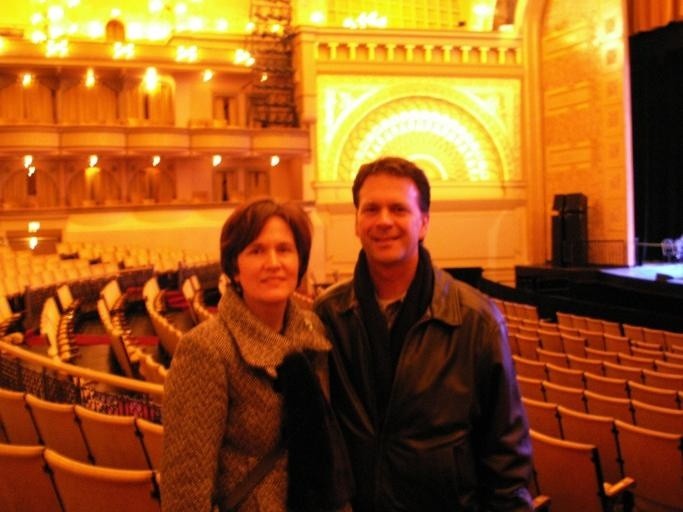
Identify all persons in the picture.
[311,157,533,512]
[159,199,335,512]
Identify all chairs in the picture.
[0,235,683,509]
